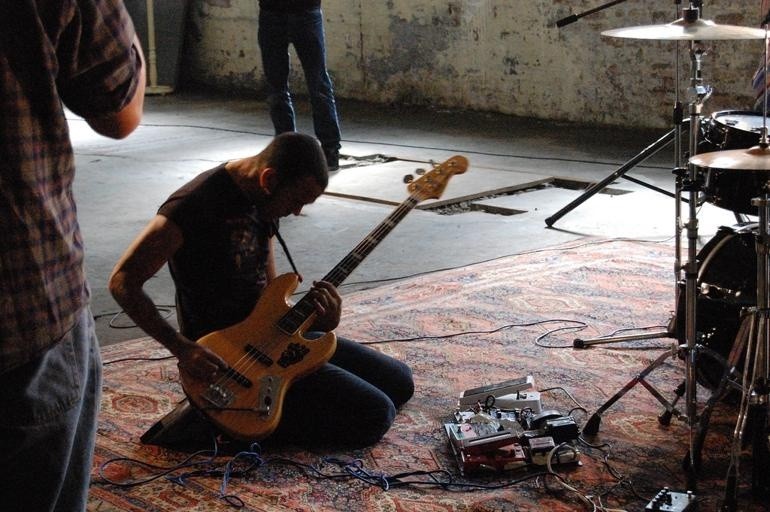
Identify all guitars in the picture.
[179,155,468,443]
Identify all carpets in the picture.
[93,233,769,510]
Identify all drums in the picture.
[667,222,770,412]
[696,110,770,216]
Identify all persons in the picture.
[255,0,342,172]
[109,132,415,452]
[0,0,149,512]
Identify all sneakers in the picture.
[139,393,191,448]
[324,149,340,171]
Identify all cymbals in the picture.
[600,18,769,40]
[688,145,770,170]
